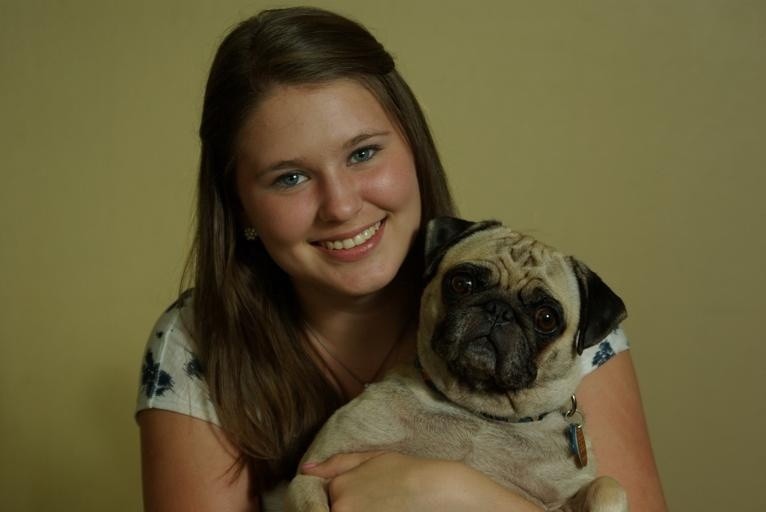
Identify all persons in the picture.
[133,7,669,512]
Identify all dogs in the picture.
[285,214,630,512]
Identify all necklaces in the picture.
[294,313,412,408]
